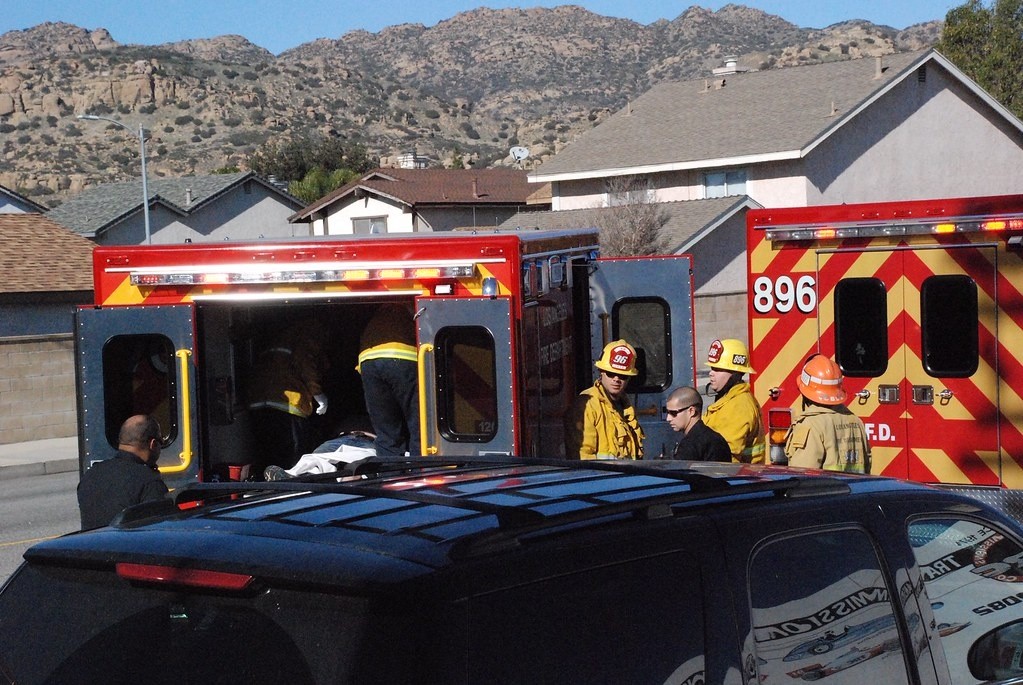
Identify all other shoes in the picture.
[264,465,295,482]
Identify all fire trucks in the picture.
[71,230,702,527]
[734,189,1022,499]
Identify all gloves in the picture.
[314,394,328,415]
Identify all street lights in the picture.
[75,113,152,246]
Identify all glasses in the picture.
[667,405,694,415]
[602,371,630,380]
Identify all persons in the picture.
[564,339,647,460]
[263,413,377,491]
[666,386,732,462]
[76,414,169,530]
[702,339,766,465]
[354,302,421,476]
[238,316,340,469]
[783,353,872,475]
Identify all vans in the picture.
[0,452,1022,685]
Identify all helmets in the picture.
[594,339,638,376]
[704,339,757,373]
[797,355,847,405]
